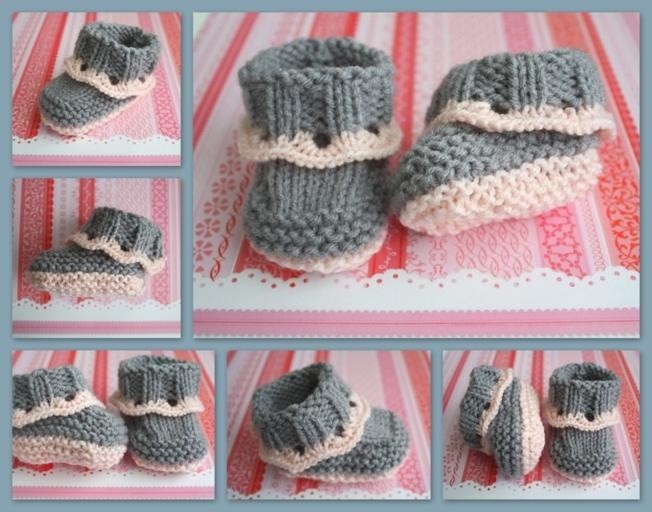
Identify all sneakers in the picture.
[13,366,129,470]
[233,36,403,273]
[545,362,621,484]
[36,23,160,137]
[460,366,547,479]
[29,206,165,296]
[392,50,617,236]
[251,362,413,484]
[109,355,210,472]
